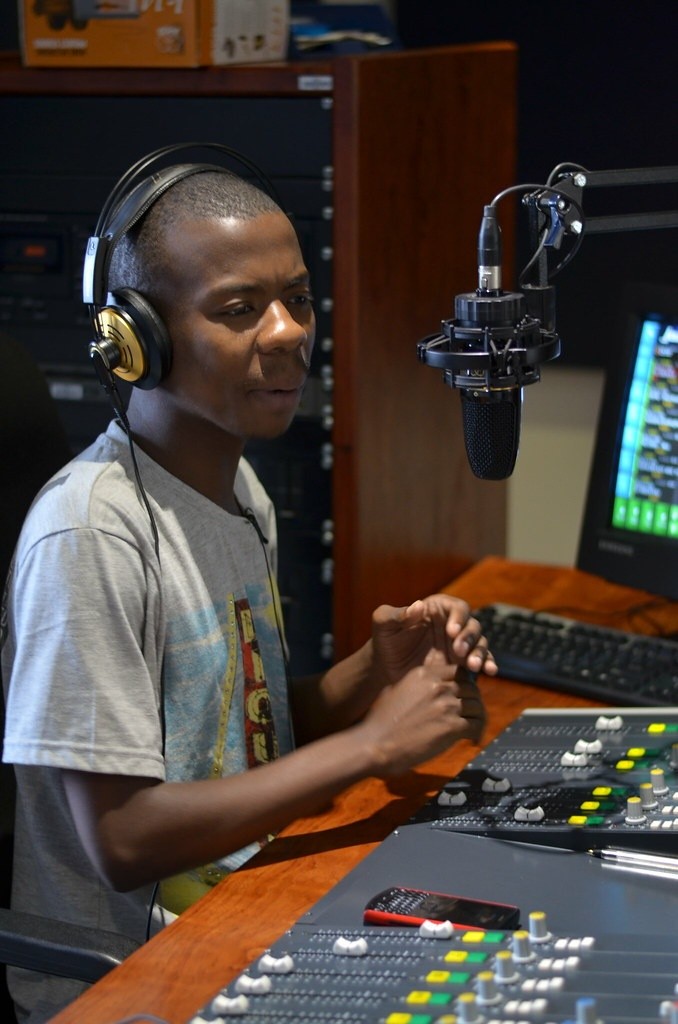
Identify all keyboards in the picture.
[469,602,678,709]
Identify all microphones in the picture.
[415,204,561,482]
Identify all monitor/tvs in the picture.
[576,286,678,599]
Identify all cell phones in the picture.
[364,884,521,934]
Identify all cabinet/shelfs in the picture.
[0,41,518,666]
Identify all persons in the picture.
[1,164,504,1024]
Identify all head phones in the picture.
[80,142,296,390]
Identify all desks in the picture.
[53,556,678,1024]
